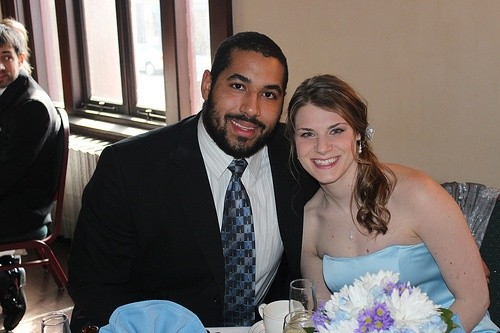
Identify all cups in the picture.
[40,313,71,333]
[258,300,305,333]
[283,310,318,333]
[289,279,315,319]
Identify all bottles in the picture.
[81,325,99,333]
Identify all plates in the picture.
[248,319,265,333]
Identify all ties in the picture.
[219,158,255,328]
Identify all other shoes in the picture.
[0,254,27,332]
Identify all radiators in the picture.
[49,132,114,239]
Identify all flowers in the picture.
[310,270,459,333]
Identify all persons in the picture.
[0,19,63,331]
[288,74,500,333]
[65,30,490,333]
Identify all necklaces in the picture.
[323,192,354,239]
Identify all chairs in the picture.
[0,100,70,287]
